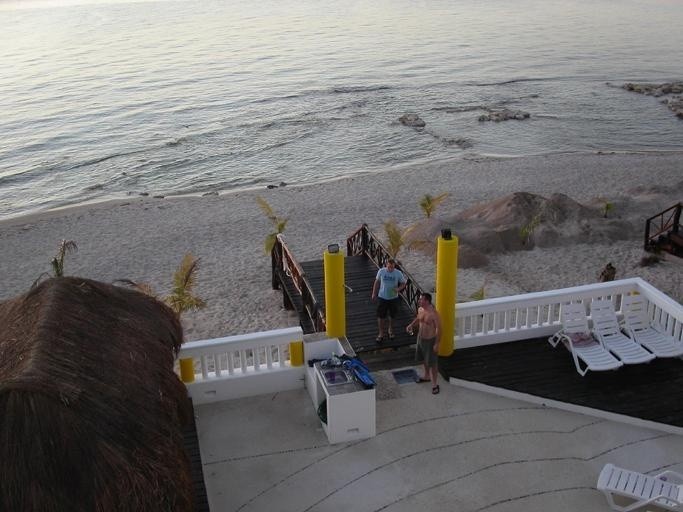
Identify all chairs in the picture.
[595,460,683,512]
[545,291,683,380]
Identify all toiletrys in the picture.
[332,351,339,365]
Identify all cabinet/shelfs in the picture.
[298,329,379,447]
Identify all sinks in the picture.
[320,367,355,386]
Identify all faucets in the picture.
[343,360,352,364]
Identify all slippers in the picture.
[388,328,395,340]
[431,384,440,395]
[376,335,384,344]
[415,377,431,383]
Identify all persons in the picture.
[370,258,406,342]
[405,292,441,394]
